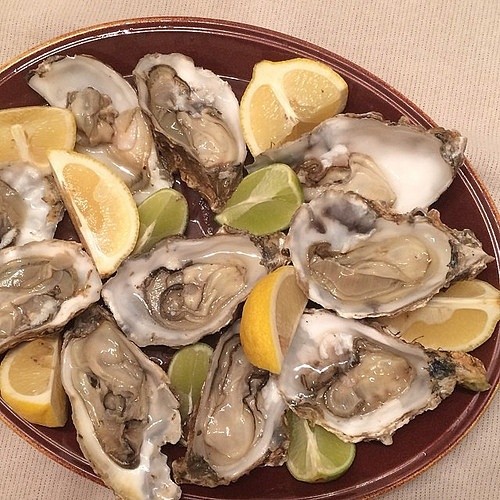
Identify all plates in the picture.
[1,15,500,500]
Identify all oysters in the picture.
[0,52,494,500]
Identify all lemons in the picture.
[0,105,77,179]
[167,342,219,445]
[239,57,349,159]
[376,277,500,353]
[285,409,356,483]
[1,337,67,427]
[214,163,305,236]
[130,188,190,258]
[240,265,308,373]
[46,147,140,279]
